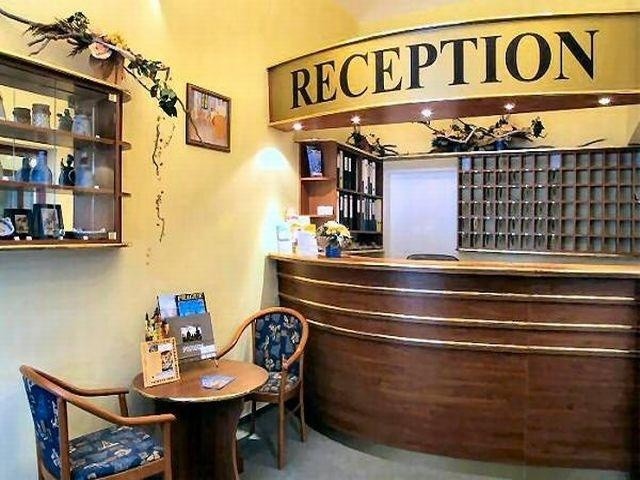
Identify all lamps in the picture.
[284,208,311,227]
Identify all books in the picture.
[159,291,216,364]
[337,148,376,231]
[307,144,323,177]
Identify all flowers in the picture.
[315,221,352,247]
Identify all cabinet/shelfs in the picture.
[458,146,640,259]
[0,48,131,252]
[298,140,385,252]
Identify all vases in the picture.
[326,245,340,257]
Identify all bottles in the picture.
[71,114,93,138]
[27,150,51,185]
[30,103,51,129]
[15,157,34,182]
[56,107,73,133]
[67,153,94,188]
[12,107,31,127]
[58,154,75,186]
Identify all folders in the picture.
[336,153,352,190]
[339,192,360,230]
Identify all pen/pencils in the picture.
[213,357,219,368]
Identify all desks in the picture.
[131,354,269,480]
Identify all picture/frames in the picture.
[34,202,64,240]
[186,82,232,151]
[300,143,326,178]
[5,207,33,241]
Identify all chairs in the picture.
[212,306,308,470]
[20,363,175,480]
[407,253,459,261]
